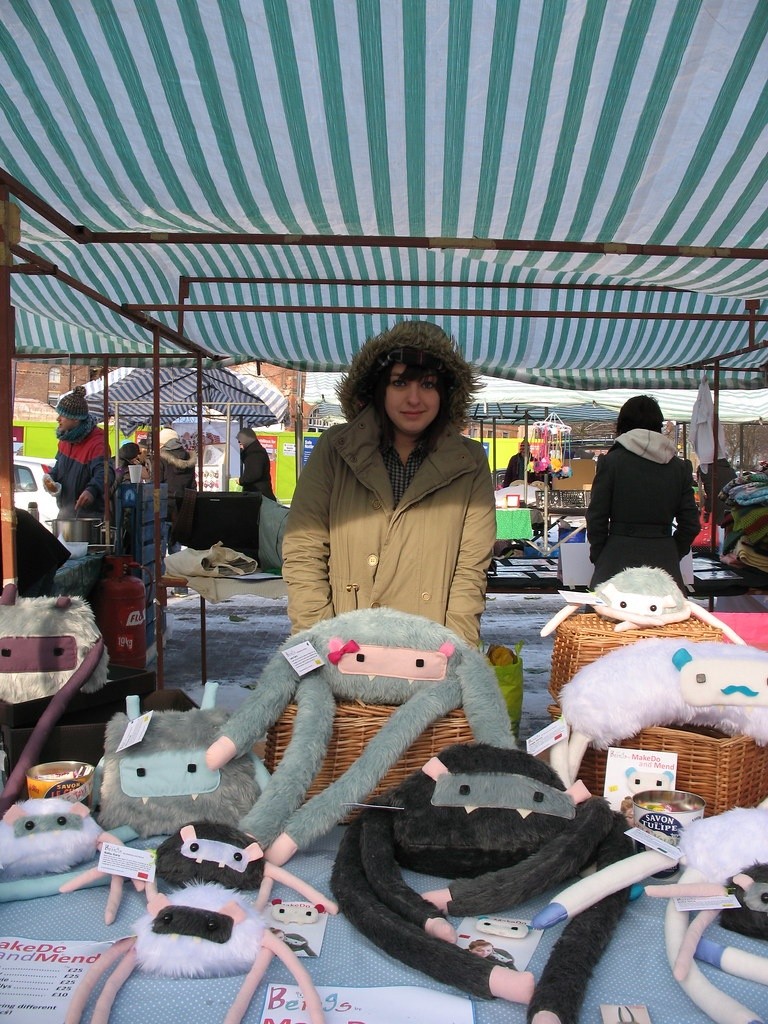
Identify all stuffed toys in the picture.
[0,566,768,1024]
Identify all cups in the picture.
[26,761,94,812]
[128,465,142,483]
[632,791,707,881]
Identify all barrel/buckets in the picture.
[558,527,586,543]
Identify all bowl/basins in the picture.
[62,541,89,558]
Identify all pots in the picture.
[45,517,105,544]
[100,526,127,554]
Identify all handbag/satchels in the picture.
[476,639,525,741]
[691,504,719,552]
[163,547,258,577]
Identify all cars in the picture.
[12,455,61,534]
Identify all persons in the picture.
[697,458,738,554]
[584,395,701,613]
[235,428,277,503]
[109,443,142,526]
[281,320,496,651]
[159,429,197,597]
[48,386,116,521]
[503,440,544,488]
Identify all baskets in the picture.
[544,704,768,819]
[536,489,592,516]
[264,697,478,824]
[548,612,722,706]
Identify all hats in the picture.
[119,442,141,462]
[55,386,90,420]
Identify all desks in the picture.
[161,553,768,684]
[539,508,588,517]
[0,822,768,1024]
[496,508,544,539]
[51,552,104,598]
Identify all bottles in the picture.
[27,502,39,522]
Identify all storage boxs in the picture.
[0,664,156,801]
